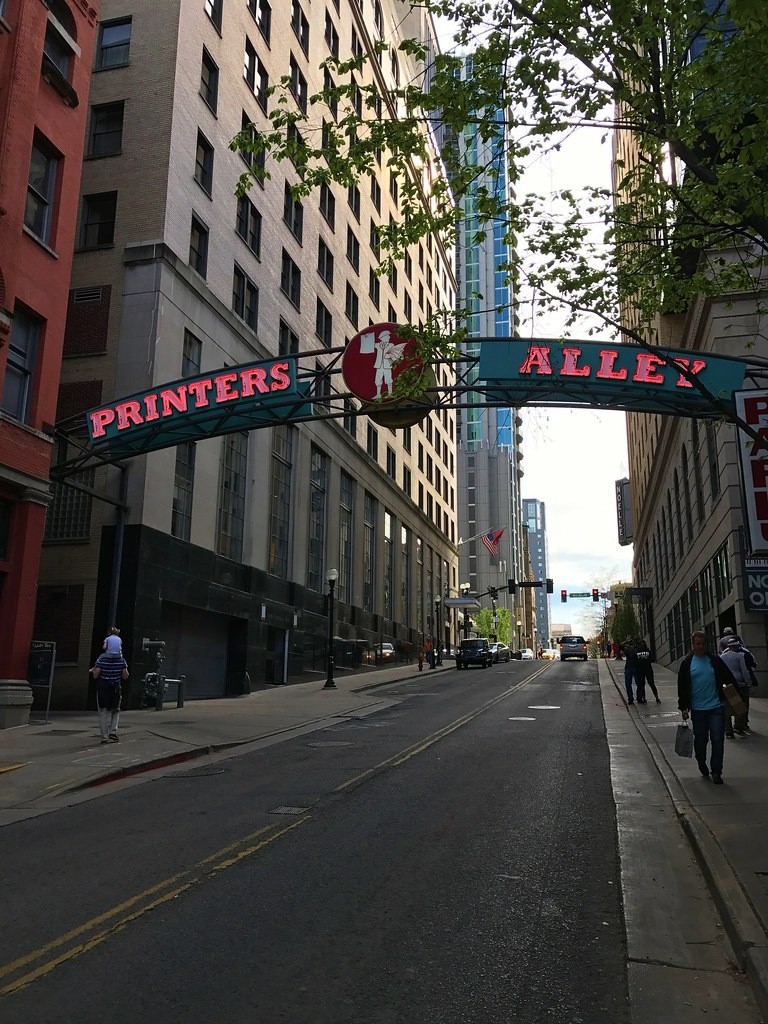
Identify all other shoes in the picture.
[628,700,633,705]
[742,728,752,736]
[100,741,107,744]
[638,700,643,703]
[107,734,119,743]
[698,760,709,777]
[643,699,647,705]
[656,699,661,704]
[726,732,735,739]
[89,668,93,672]
[710,771,724,784]
[733,728,746,736]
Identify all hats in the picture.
[723,627,734,635]
[727,638,742,646]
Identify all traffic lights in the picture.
[560,590,567,603]
[494,588,499,600]
[592,589,599,603]
[490,586,496,598]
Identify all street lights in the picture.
[459,582,470,640]
[322,567,339,691]
[517,621,522,650]
[534,628,539,660]
[434,594,443,667]
[613,600,624,660]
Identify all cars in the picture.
[542,649,560,660]
[376,643,395,660]
[521,648,533,660]
[488,642,511,664]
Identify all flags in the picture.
[482,531,498,557]
[493,530,504,545]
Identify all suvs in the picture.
[455,638,493,670]
[558,636,590,661]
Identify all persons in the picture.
[425,639,433,663]
[607,641,611,658]
[538,645,543,659]
[619,635,661,705]
[719,627,757,739]
[677,631,739,785]
[88,627,129,743]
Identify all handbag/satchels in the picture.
[675,719,693,758]
